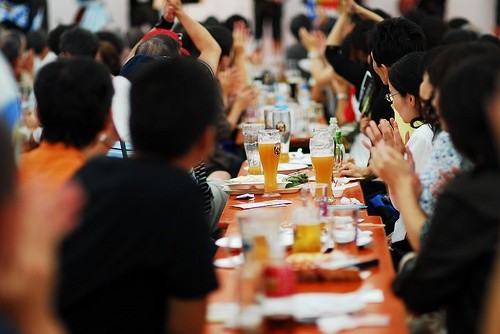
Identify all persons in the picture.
[0,0,500,334]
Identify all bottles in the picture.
[334,132,345,167]
[246,80,312,134]
[222,174,292,195]
[243,236,297,327]
[329,116,341,153]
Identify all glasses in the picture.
[385,92,401,102]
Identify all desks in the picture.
[234,107,328,147]
[218,159,369,228]
[211,215,410,334]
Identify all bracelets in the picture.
[155,15,175,29]
[308,50,318,58]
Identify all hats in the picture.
[143,29,189,56]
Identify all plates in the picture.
[215,235,242,250]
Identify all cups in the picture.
[237,108,357,260]
